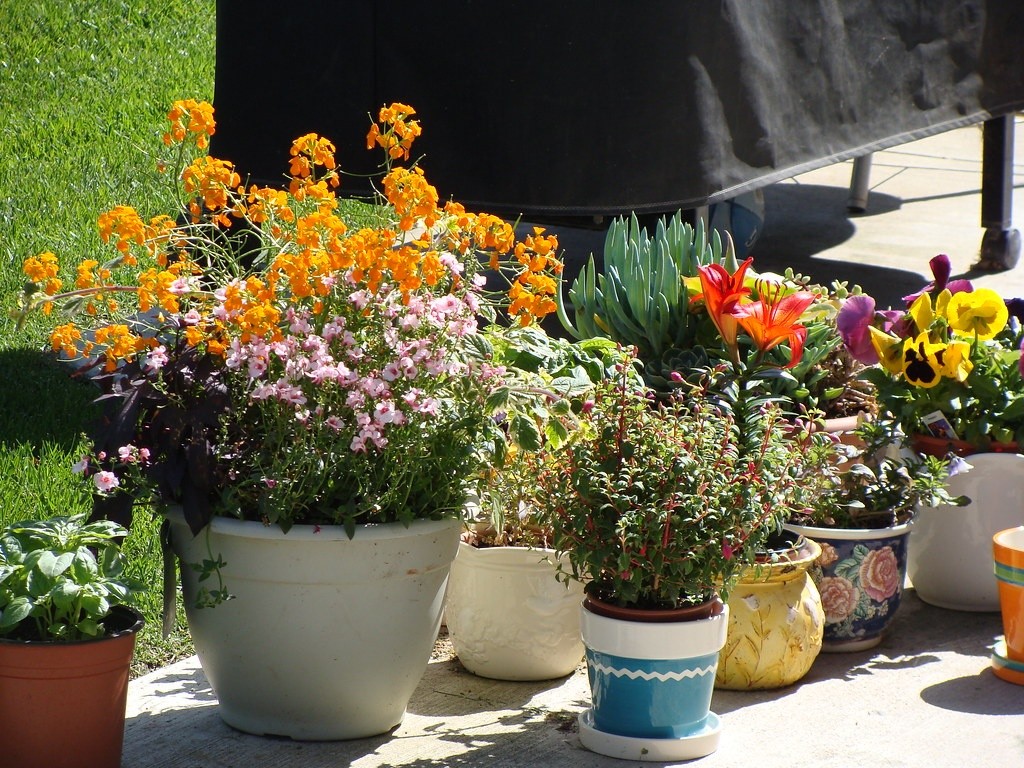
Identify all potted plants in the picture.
[443,328,644,685]
[0,512,145,768]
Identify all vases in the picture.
[785,527,918,654]
[575,595,729,762]
[713,528,825,692]
[905,454,1024,612]
[990,524,1024,685]
[161,508,464,739]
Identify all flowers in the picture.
[836,253,1024,450]
[671,257,821,544]
[516,343,841,604]
[22,96,561,537]
[849,421,978,525]
